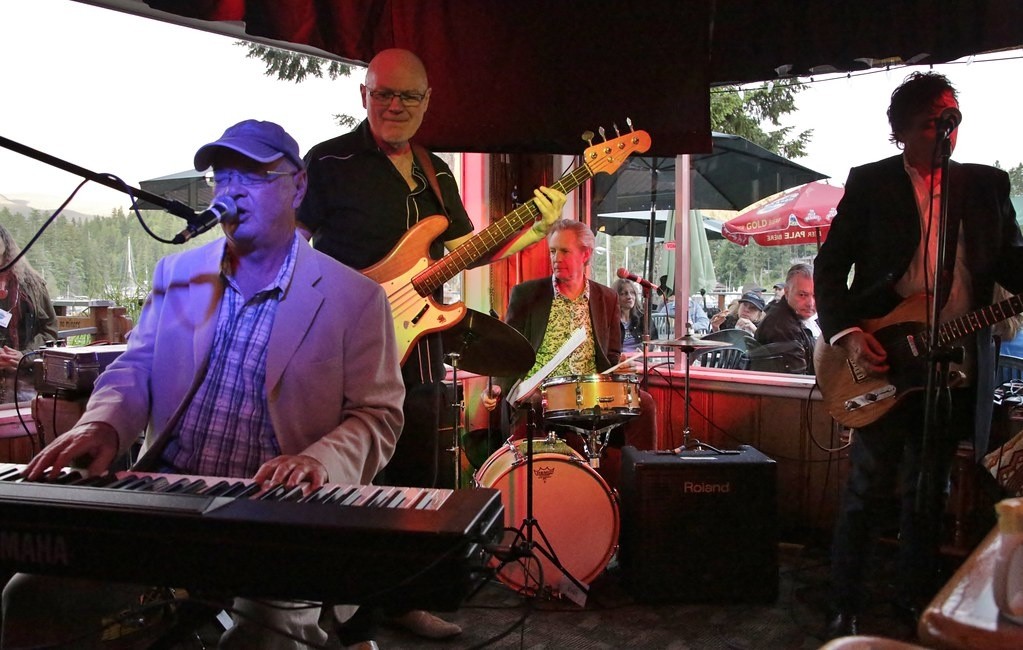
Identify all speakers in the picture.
[618,444,779,602]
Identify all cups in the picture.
[993,499,1023,621]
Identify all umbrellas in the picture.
[657,209,716,299]
[722,178,848,253]
[561,132,831,335]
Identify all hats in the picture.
[194,118,305,172]
[773,283,784,289]
[753,288,766,292]
[738,291,765,312]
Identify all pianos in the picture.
[0,459,507,650]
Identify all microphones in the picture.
[936,107,962,140]
[617,268,662,291]
[172,194,237,245]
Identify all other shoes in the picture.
[338,638,379,650]
[390,606,463,642]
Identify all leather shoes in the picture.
[822,589,859,644]
[889,593,927,633]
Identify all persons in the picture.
[709,281,787,338]
[659,300,710,333]
[610,278,659,351]
[745,264,817,375]
[814,72,1022,642]
[295,47,567,650]
[481,219,656,452]
[0,226,58,403]
[0,119,407,650]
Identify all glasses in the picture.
[365,85,425,107]
[204,165,298,187]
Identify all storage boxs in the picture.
[42,344,130,389]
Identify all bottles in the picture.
[652,346,661,364]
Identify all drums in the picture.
[540,371,643,421]
[470,432,623,595]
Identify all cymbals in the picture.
[643,337,734,349]
[420,303,537,378]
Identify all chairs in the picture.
[651,308,787,373]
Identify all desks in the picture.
[917,518,1023,650]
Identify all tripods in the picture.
[464,327,605,610]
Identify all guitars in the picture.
[813,293,1023,428]
[358,117,652,368]
